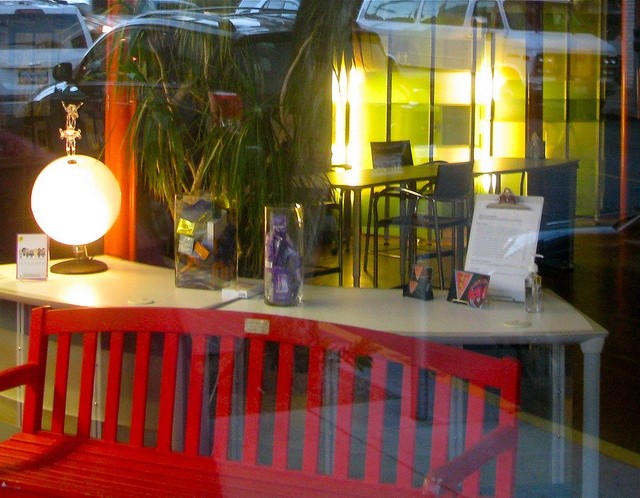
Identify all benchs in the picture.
[1,306,522,496]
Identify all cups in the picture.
[171,190,240,291]
[262,202,305,306]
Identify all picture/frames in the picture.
[16,234,49,280]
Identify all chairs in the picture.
[363,140,433,274]
[373,186,468,290]
[304,189,345,286]
[412,161,475,281]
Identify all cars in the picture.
[234,1,299,17]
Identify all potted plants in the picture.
[85,0,363,355]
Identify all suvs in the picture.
[23,6,404,190]
[0,0,93,123]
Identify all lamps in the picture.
[31,101,122,275]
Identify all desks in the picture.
[292,158,582,288]
[0,255,610,498]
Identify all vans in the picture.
[356,0,621,122]
